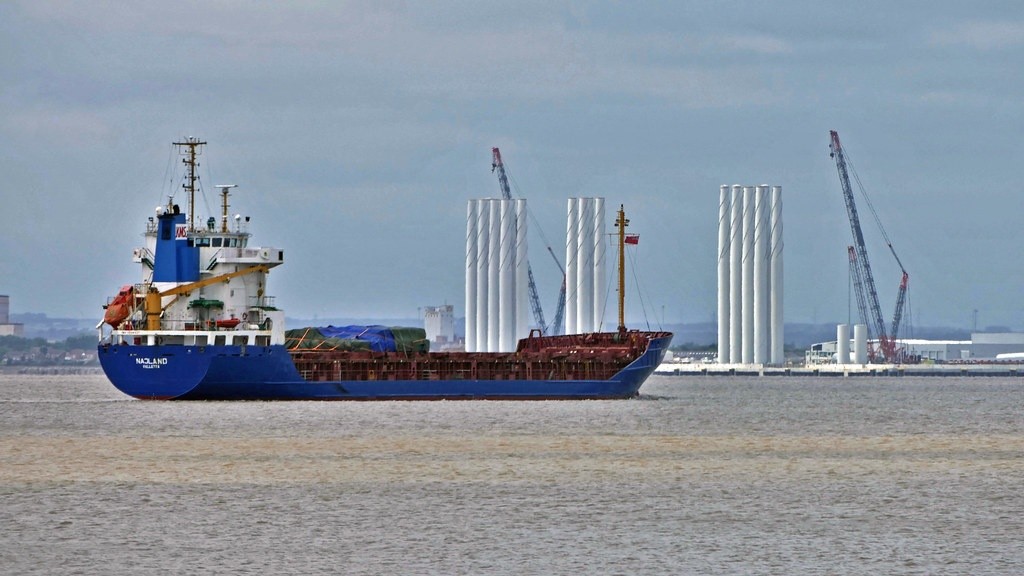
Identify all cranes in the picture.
[489,146,567,337]
[829,130,909,359]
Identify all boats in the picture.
[96,135,674,400]
